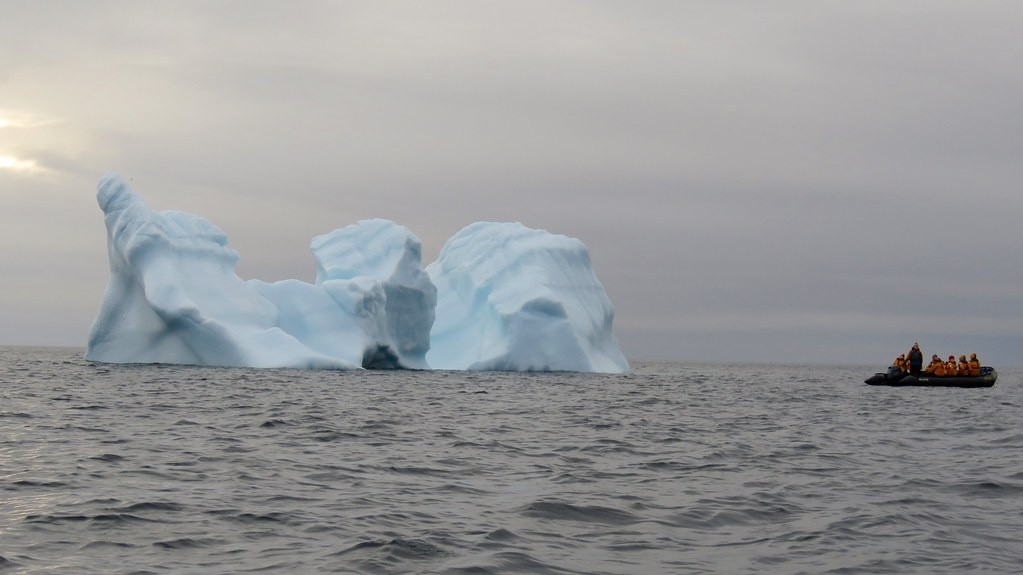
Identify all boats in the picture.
[864,366,998,388]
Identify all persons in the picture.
[925,354,981,377]
[902,342,923,377]
[893,354,905,373]
